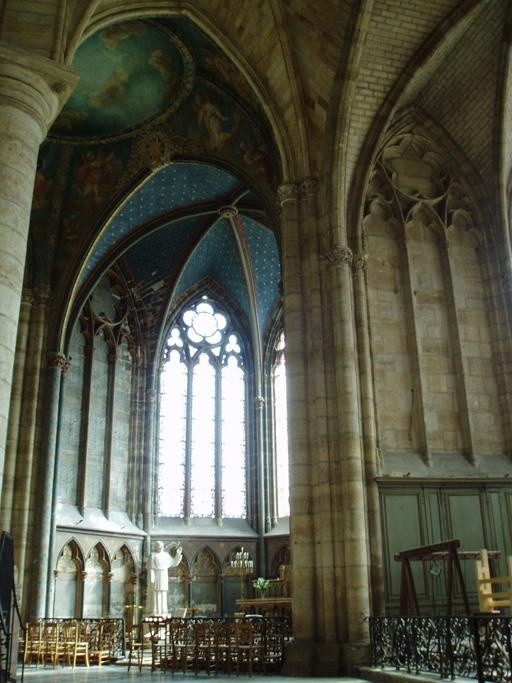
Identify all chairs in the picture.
[22,619,117,669]
[127,617,254,677]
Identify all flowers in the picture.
[252,577,272,593]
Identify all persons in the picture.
[148,541,183,613]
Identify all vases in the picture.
[260,588,266,600]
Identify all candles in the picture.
[230,546,254,568]
